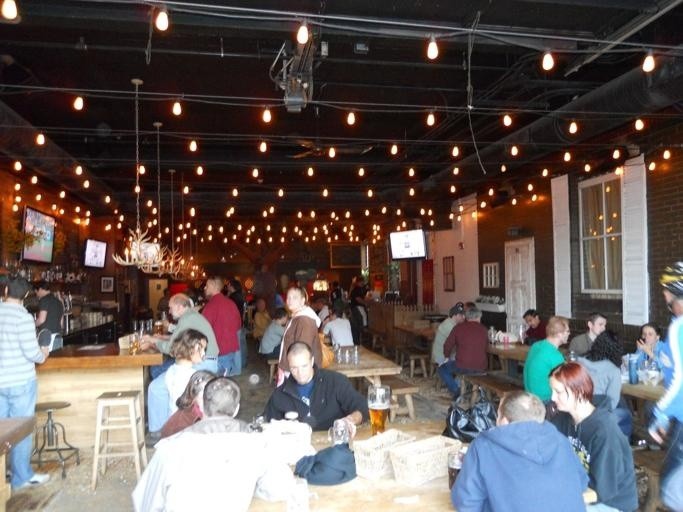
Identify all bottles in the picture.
[567,350,576,362]
[56,289,71,312]
[641,360,660,389]
[161,312,169,336]
[128,318,154,352]
[333,343,360,364]
[489,326,495,344]
[2,256,64,281]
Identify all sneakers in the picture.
[16,474,50,490]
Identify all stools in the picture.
[34,402,79,478]
[90,391,147,490]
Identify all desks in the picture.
[0,417,39,512]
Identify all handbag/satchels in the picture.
[442,387,496,442]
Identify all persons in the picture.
[132,378,295,512]
[0,276,49,491]
[647,260,683,512]
[136,276,374,446]
[32,282,64,352]
[432,301,664,511]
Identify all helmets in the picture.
[658,260,683,295]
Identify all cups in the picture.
[325,421,349,444]
[629,358,638,384]
[284,411,299,422]
[516,322,526,345]
[447,450,463,489]
[155,320,163,335]
[503,336,508,343]
[367,385,389,435]
[252,412,267,433]
[80,311,113,323]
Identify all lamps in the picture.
[642,50,655,73]
[296,24,309,45]
[426,39,438,60]
[1,1,18,22]
[155,11,170,32]
[0,92,671,276]
[542,53,555,71]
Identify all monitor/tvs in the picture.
[81,237,108,269]
[19,204,58,266]
[330,243,362,269]
[389,229,427,260]
[130,240,163,268]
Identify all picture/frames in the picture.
[101,277,114,293]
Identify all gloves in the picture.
[646,414,670,450]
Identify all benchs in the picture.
[255,324,683,511]
